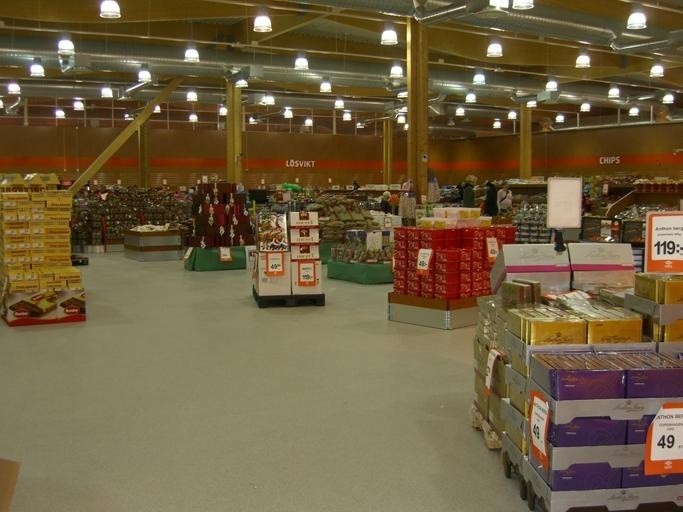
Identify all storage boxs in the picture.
[393,226,516,299]
[0,190,83,292]
[532,350,683,492]
[472,274,683,456]
[418,207,492,229]
[184,182,254,248]
[487,244,637,294]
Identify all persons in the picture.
[496,181,513,212]
[486,182,498,216]
[463,174,478,207]
[388,192,398,216]
[380,191,391,214]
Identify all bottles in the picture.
[664,261,672,281]
